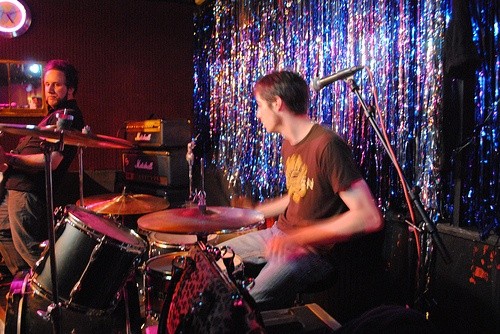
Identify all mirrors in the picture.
[0,59,48,117]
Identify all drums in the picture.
[29,205,147,318]
[142,252,243,321]
[147,229,219,257]
[4,270,131,334]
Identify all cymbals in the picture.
[77,192,170,214]
[137,205,267,234]
[0,123,129,150]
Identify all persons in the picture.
[214,71,383,301]
[0,60,88,274]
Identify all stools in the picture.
[281,270,333,305]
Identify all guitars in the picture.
[0,150,20,204]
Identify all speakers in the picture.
[121,117,191,209]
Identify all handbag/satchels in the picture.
[156,245,264,334]
[167,240,264,334]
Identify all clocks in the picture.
[0,0,32,38]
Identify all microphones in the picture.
[309,66,363,91]
